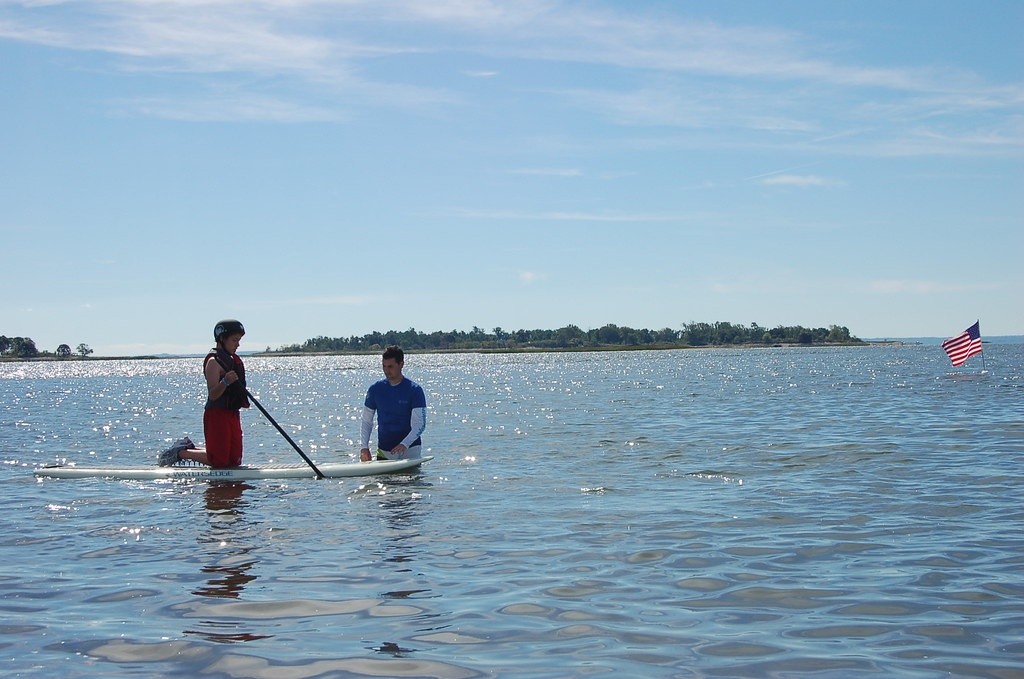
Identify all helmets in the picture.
[214,320,245,341]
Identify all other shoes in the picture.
[170,436,195,449]
[158,445,187,467]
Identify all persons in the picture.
[159,318,251,468]
[361,346,426,461]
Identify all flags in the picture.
[943,322,982,367]
